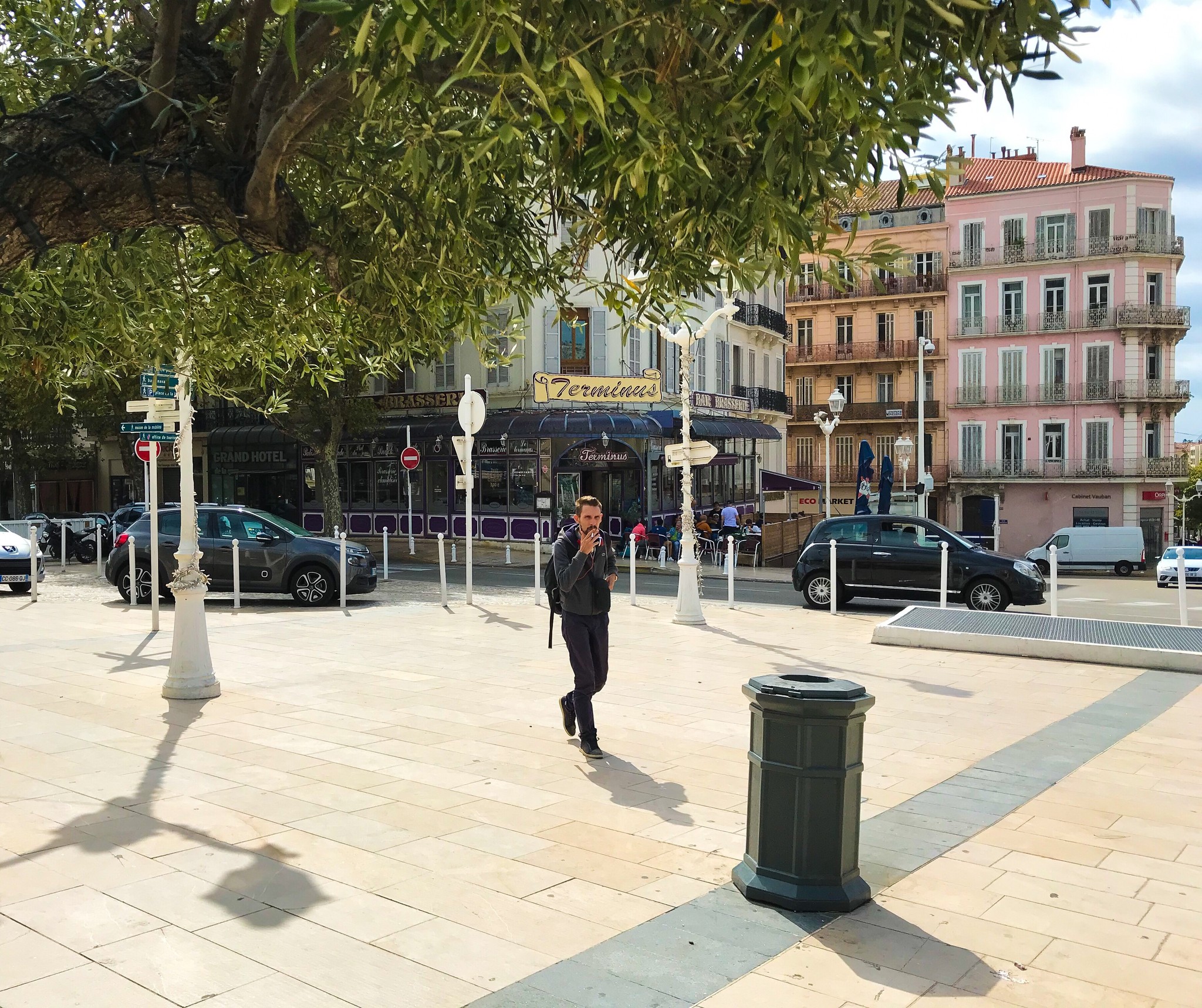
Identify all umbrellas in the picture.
[854,440,875,541]
[877,456,894,516]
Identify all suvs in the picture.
[791,514,1048,613]
[19,502,269,556]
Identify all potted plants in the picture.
[732,533,761,565]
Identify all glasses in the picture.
[714,517,719,518]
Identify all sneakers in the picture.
[579,738,604,758]
[559,696,575,737]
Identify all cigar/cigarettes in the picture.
[591,533,593,538]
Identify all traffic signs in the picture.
[121,423,164,432]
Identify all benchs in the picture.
[827,530,856,541]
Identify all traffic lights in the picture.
[131,439,143,467]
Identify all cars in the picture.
[105,506,377,607]
[1154,546,1202,588]
[0,523,45,593]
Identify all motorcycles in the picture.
[37,517,97,565]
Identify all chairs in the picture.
[886,530,899,546]
[622,521,773,569]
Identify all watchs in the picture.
[614,574,617,582]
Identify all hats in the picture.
[727,500,733,504]
[713,514,719,516]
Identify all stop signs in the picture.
[134,438,161,462]
[401,447,419,470]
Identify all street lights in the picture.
[621,251,744,626]
[1165,478,1202,547]
[812,388,846,516]
[894,437,915,496]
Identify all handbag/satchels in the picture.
[590,578,611,612]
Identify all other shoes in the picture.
[667,557,674,562]
[640,555,645,559]
[648,556,657,560]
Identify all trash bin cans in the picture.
[733,673,872,914]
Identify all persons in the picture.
[882,527,920,546]
[791,510,805,519]
[1197,536,1202,545]
[553,495,618,757]
[632,500,767,566]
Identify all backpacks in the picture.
[544,531,612,615]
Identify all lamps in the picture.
[436,434,444,449]
[602,431,609,448]
[499,433,508,447]
[371,437,379,450]
[756,453,762,464]
[737,453,743,464]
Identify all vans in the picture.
[1024,527,1146,577]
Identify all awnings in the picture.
[207,409,663,445]
[674,417,783,440]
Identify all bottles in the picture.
[746,526,748,534]
